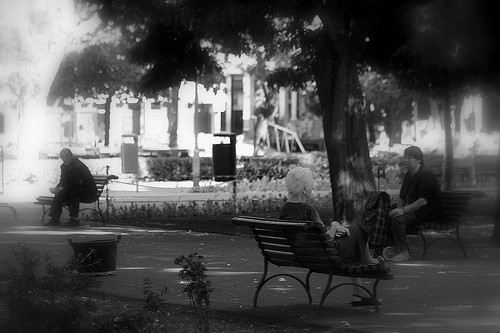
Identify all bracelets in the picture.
[402,206,406,214]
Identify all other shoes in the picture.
[43,219,61,226]
[63,218,81,226]
[391,251,410,262]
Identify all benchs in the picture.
[142,148,205,158]
[35,174,119,225]
[405,189,486,264]
[231,215,392,317]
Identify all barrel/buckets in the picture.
[68,232,123,277]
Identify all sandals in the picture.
[350,293,382,307]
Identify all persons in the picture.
[279,166,380,268]
[44,148,98,227]
[385,145,444,262]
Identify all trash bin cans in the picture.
[211,131,237,182]
[120,132,139,173]
